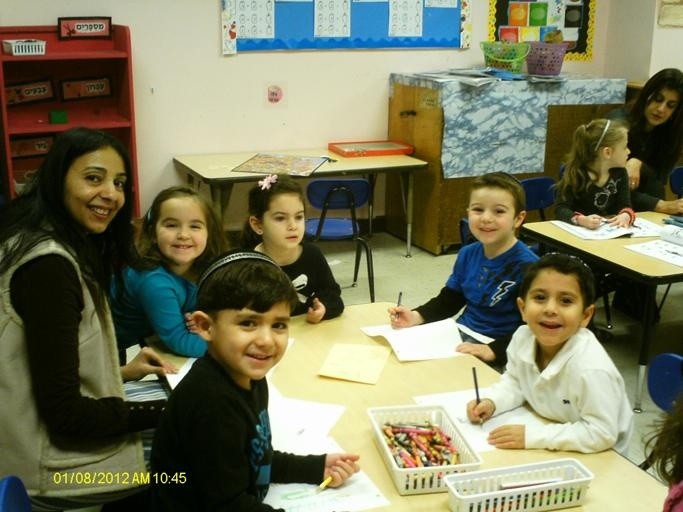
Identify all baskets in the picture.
[523,40,569,75]
[479,40,530,73]
[366,405,480,496]
[442,458,594,512]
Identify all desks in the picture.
[169,140,432,260]
[141,300,670,511]
[518,208,683,415]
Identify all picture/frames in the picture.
[325,138,419,160]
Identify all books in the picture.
[550,216,660,240]
[623,224,683,268]
[413,66,567,88]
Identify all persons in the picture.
[109,184,230,360]
[1,123,178,511]
[465,250,645,461]
[552,117,636,229]
[242,171,345,324]
[149,247,363,512]
[642,391,683,512]
[606,67,683,215]
[387,170,540,367]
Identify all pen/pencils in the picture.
[470,478,591,512]
[383,421,465,480]
[472,367,483,428]
[396,292,402,320]
[315,477,332,493]
[574,211,609,224]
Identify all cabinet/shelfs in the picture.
[382,67,632,259]
[0,20,142,246]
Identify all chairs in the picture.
[0,476,34,512]
[634,351,683,473]
[509,177,613,332]
[656,166,683,316]
[458,215,479,250]
[295,178,375,303]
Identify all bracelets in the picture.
[618,207,635,225]
[571,210,584,225]
